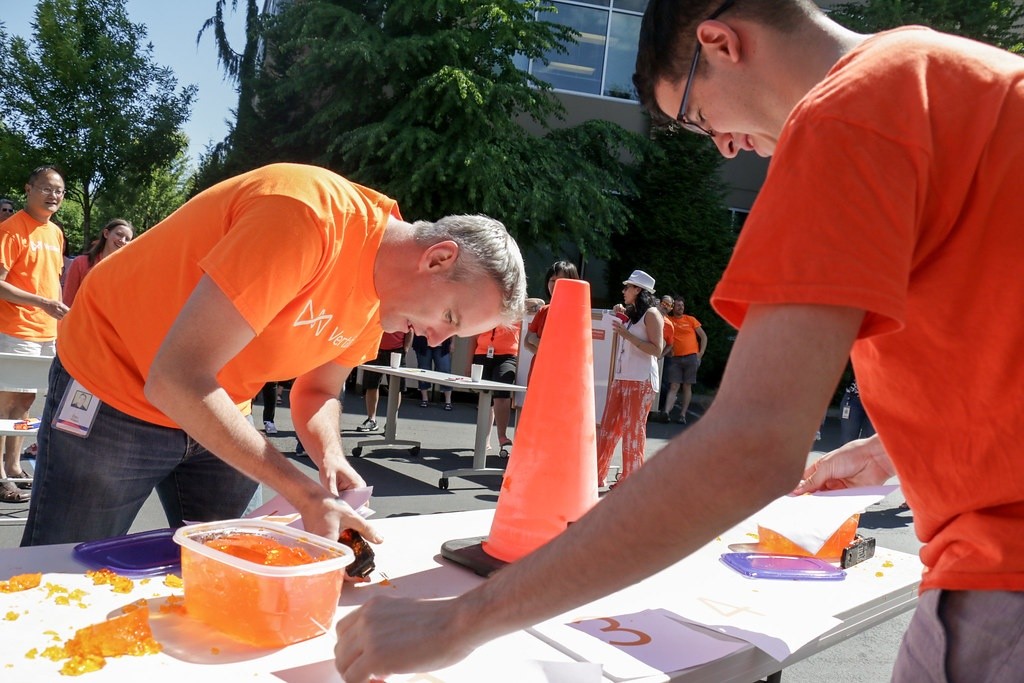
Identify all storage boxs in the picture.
[173,519,356,647]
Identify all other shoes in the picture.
[445,403,453,412]
[275,393,282,403]
[421,400,429,409]
[499,436,513,446]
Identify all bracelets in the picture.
[697,353,703,358]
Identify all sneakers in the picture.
[264,421,277,433]
[356,418,378,432]
[678,414,688,424]
[659,412,671,424]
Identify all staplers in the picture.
[338,529,375,578]
[840,533,875,569]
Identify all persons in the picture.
[649,293,707,425]
[597,266,666,491]
[523,260,579,391]
[24,218,140,460]
[334,0,1024,683]
[19,162,527,550]
[0,164,72,503]
[262,329,456,437]
[839,378,876,448]
[0,199,14,222]
[71,393,88,411]
[465,298,546,451]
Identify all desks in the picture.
[0,511,925,683]
[352,365,528,490]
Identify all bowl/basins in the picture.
[172,518,356,650]
[759,513,859,562]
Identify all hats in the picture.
[623,270,656,294]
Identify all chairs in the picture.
[0,353,55,524]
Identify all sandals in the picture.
[7,469,34,489]
[0,481,30,501]
[24,444,39,454]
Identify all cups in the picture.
[390,352,402,369]
[471,364,483,382]
[616,312,629,326]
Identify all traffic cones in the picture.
[439,278,599,578]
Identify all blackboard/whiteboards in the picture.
[511,308,618,428]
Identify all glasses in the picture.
[668,0,736,136]
[29,183,66,197]
[0,207,13,214]
[662,301,673,308]
[624,286,638,290]
